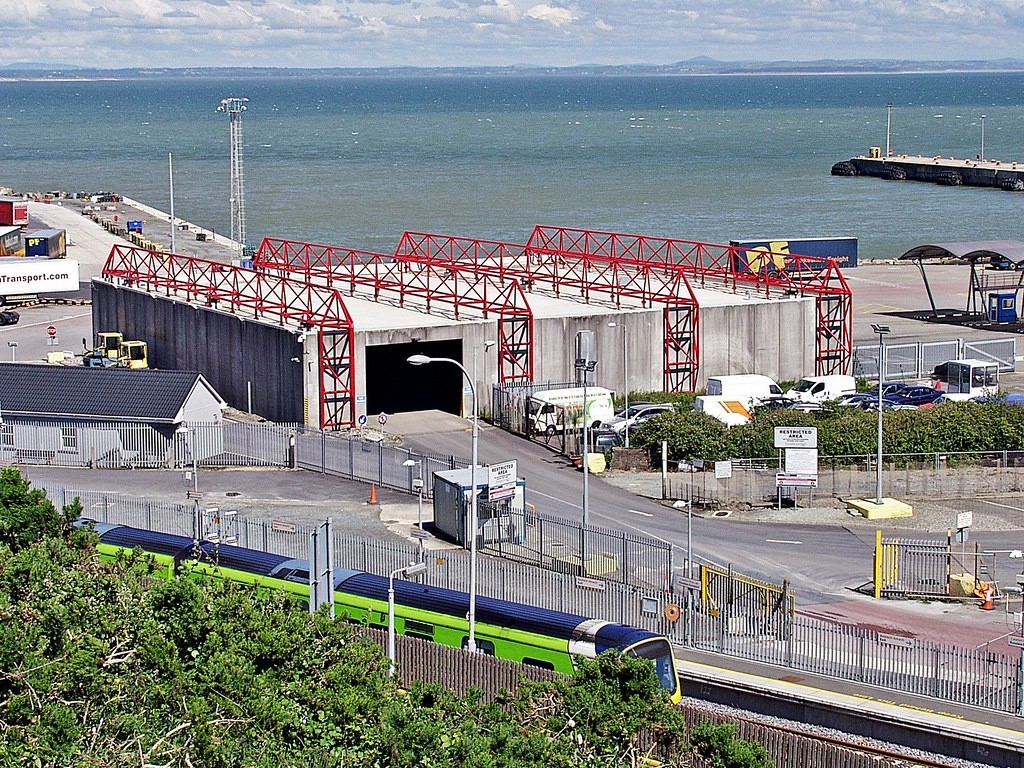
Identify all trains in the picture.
[65,521,686,709]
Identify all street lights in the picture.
[870,322,892,510]
[405,353,488,661]
[572,329,597,577]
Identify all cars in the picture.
[792,382,943,408]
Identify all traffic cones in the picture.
[978,583,996,610]
[934,379,942,390]
[367,482,379,504]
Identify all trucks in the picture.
[694,393,791,431]
[791,376,859,400]
[601,403,674,432]
[0,257,81,301]
[0,198,29,228]
[707,374,781,398]
[524,387,617,438]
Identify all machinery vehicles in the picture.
[81,333,152,372]
